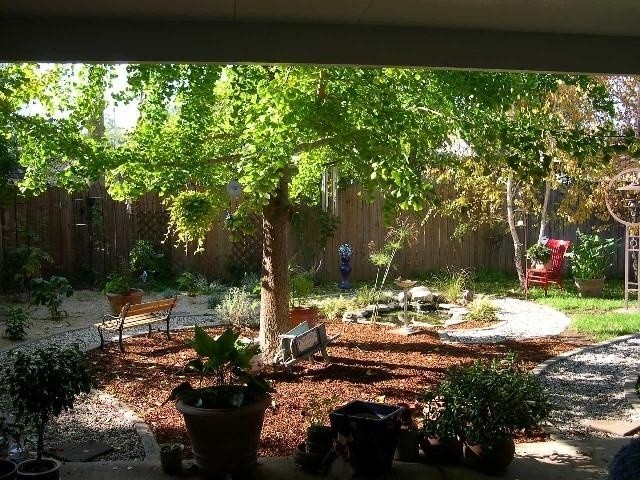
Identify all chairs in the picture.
[519,236,573,299]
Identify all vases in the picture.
[1,460,18,479]
[335,256,357,291]
[328,397,405,479]
[287,306,319,331]
[396,401,463,468]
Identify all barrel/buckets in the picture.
[240,339,263,372]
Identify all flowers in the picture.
[337,243,354,263]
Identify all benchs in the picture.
[93,295,179,357]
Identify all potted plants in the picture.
[104,269,145,317]
[525,241,556,271]
[169,322,280,472]
[1,339,98,480]
[562,228,621,298]
[298,388,342,454]
[424,350,558,476]
[159,441,185,475]
